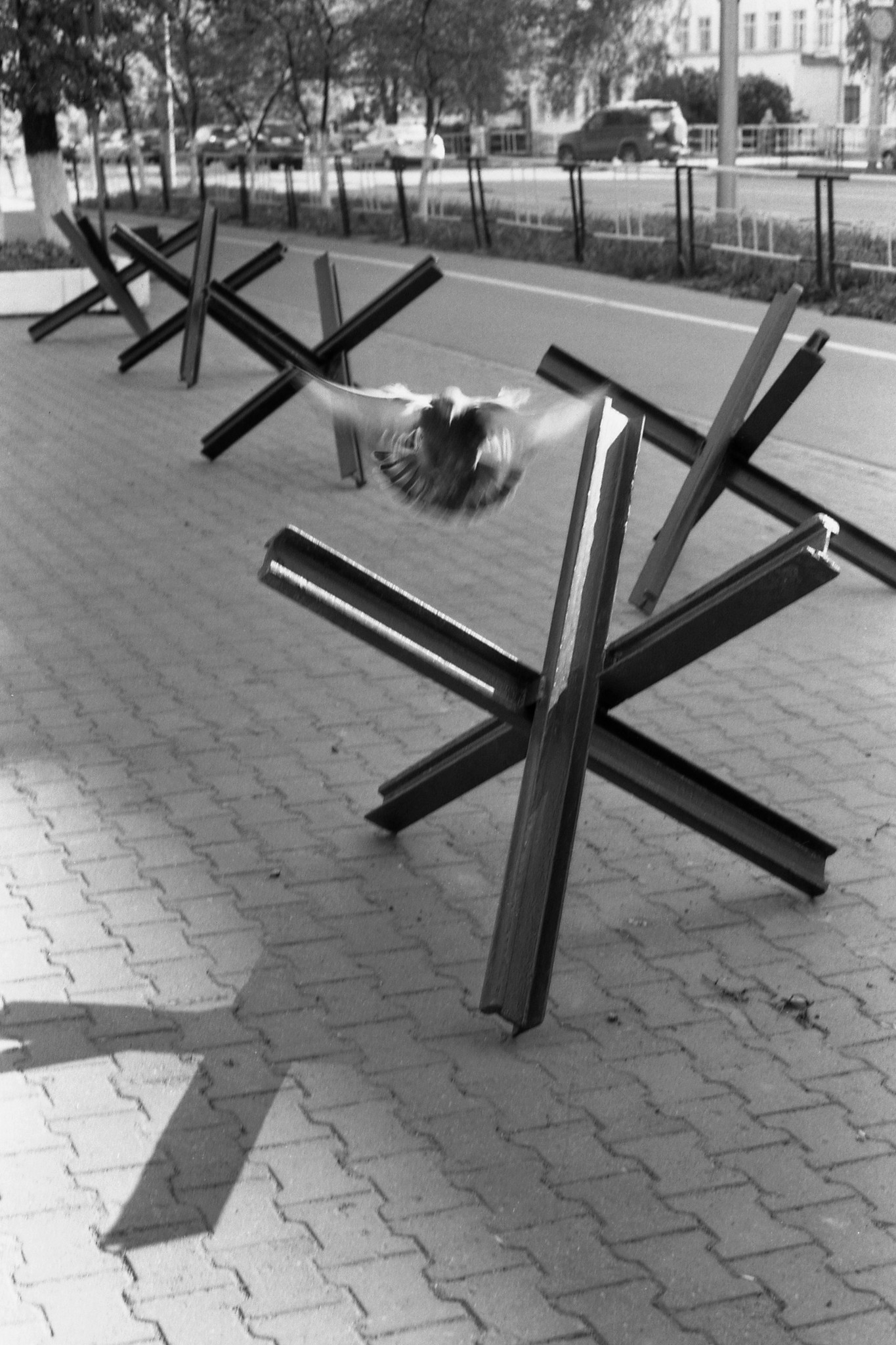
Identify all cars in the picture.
[58,126,163,164]
[224,116,304,170]
[348,122,446,173]
[189,123,239,165]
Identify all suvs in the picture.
[554,97,688,172]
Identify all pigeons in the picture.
[272,350,618,528]
